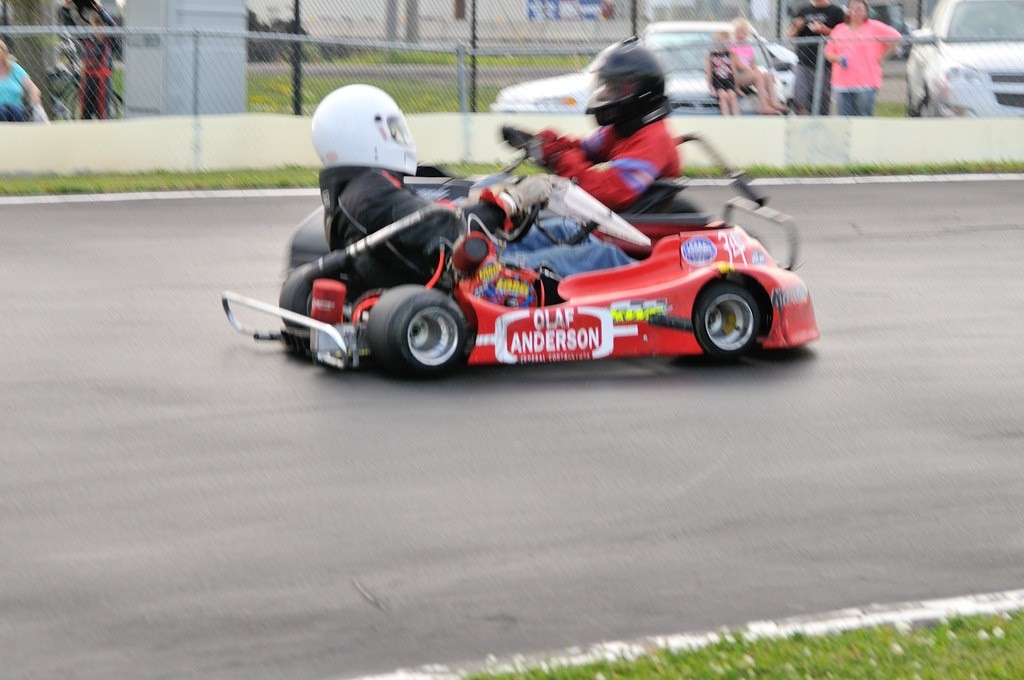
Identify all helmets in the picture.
[313,81,419,175]
[581,37,673,133]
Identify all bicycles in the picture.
[38,22,124,125]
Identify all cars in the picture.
[638,18,784,119]
[903,0,1024,120]
[491,33,803,119]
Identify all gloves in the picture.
[500,127,543,162]
[504,172,553,212]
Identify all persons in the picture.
[728,19,790,114]
[62,0,124,119]
[788,0,848,116]
[823,1,903,117]
[703,31,747,114]
[1,41,46,121]
[311,83,642,312]
[474,38,685,212]
